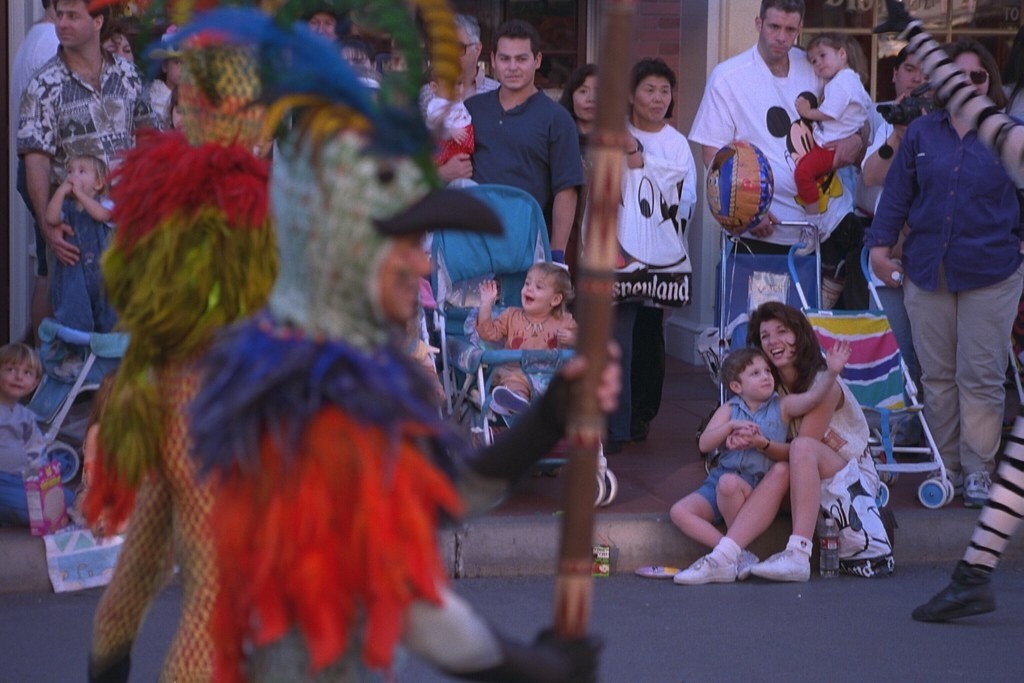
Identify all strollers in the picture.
[690,220,824,478]
[429,183,620,508]
[786,228,957,509]
[24,318,131,486]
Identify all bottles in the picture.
[820,518,840,578]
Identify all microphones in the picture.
[876,104,892,113]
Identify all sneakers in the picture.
[961,473,990,505]
[929,466,963,495]
[751,553,811,582]
[673,550,736,584]
[795,225,830,255]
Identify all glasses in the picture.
[968,69,990,84]
[457,41,477,56]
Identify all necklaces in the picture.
[523,311,552,335]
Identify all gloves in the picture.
[875,0,920,32]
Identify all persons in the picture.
[0,1,1024,683]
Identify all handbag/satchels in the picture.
[818,458,896,575]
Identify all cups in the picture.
[821,271,846,311]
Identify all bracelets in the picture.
[757,437,771,452]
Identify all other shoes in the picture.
[911,562,996,620]
[737,551,759,580]
[492,385,530,413]
